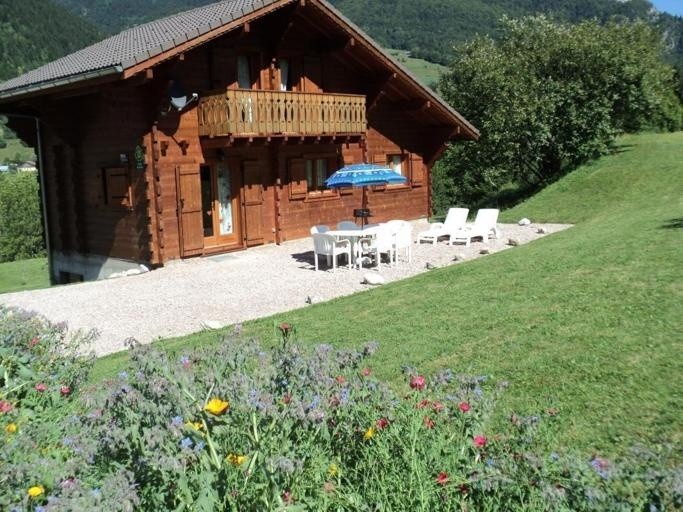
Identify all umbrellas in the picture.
[325,163,407,230]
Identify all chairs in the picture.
[449,208,499,246]
[310,219,411,275]
[416,208,469,244]
